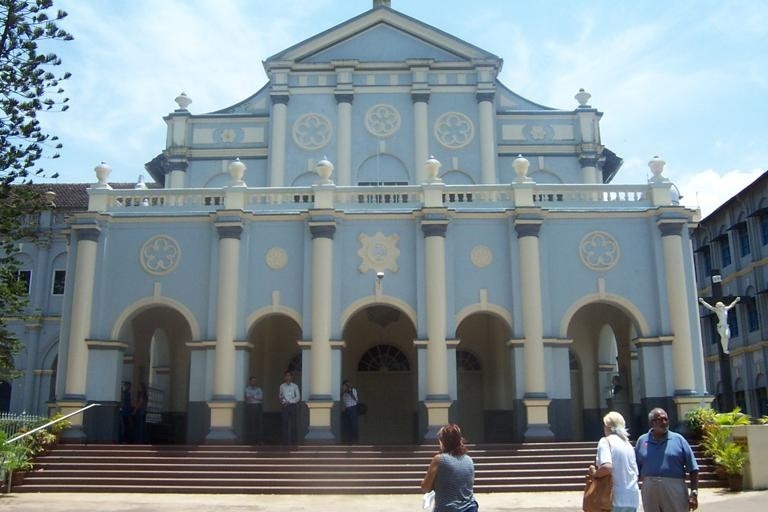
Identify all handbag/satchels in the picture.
[583,465,612,509]
[358,403,368,415]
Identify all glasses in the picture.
[652,418,668,421]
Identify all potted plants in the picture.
[688,409,746,491]
[2,414,68,487]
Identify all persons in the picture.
[245,377,263,446]
[421,423,479,512]
[342,380,359,443]
[117,382,146,444]
[698,297,740,355]
[634,407,699,512]
[278,371,301,445]
[588,411,640,512]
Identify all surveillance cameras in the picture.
[376,272,384,278]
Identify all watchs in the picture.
[689,490,698,498]
